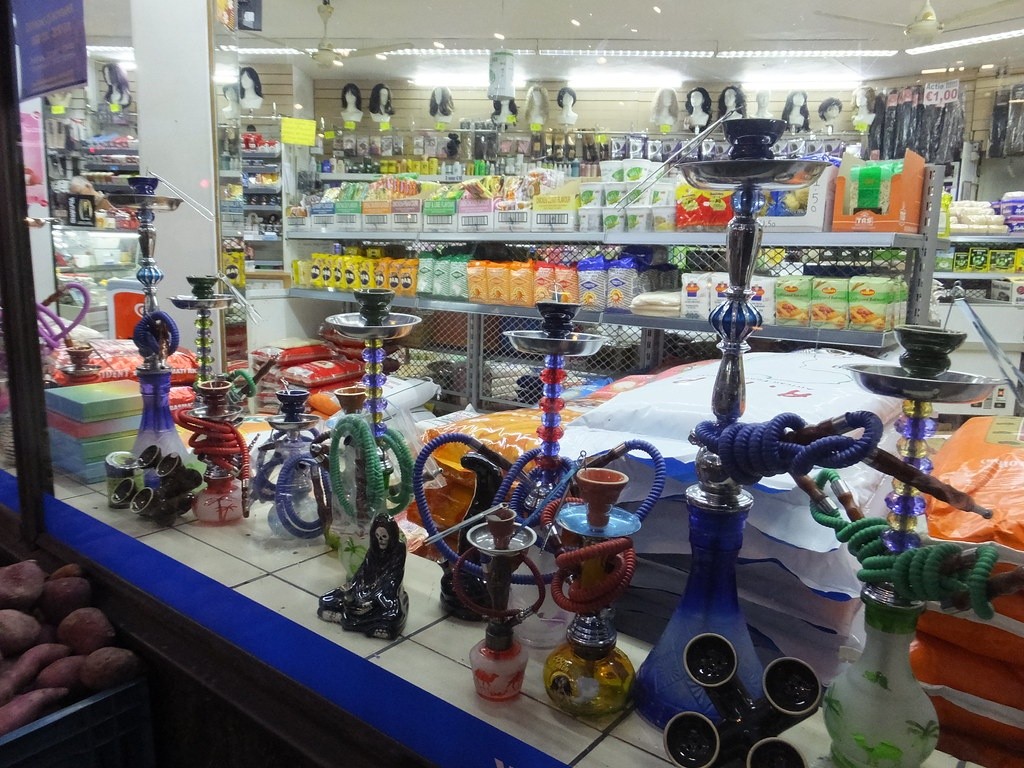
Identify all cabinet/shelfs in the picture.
[283,120,1024,420]
[48,125,281,272]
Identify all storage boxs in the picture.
[831,148,925,235]
[836,248,854,267]
[1016,248,1024,274]
[802,249,819,266]
[933,246,955,272]
[756,249,785,276]
[969,246,988,274]
[872,250,915,278]
[953,253,968,272]
[0,685,150,768]
[669,246,708,268]
[752,163,837,234]
[854,248,871,268]
[785,251,803,276]
[686,250,724,272]
[44,379,144,485]
[988,251,1017,274]
[819,248,836,266]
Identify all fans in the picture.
[239,1,414,70]
[815,0,1024,41]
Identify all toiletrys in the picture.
[306,153,599,178]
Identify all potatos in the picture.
[0,559,138,736]
